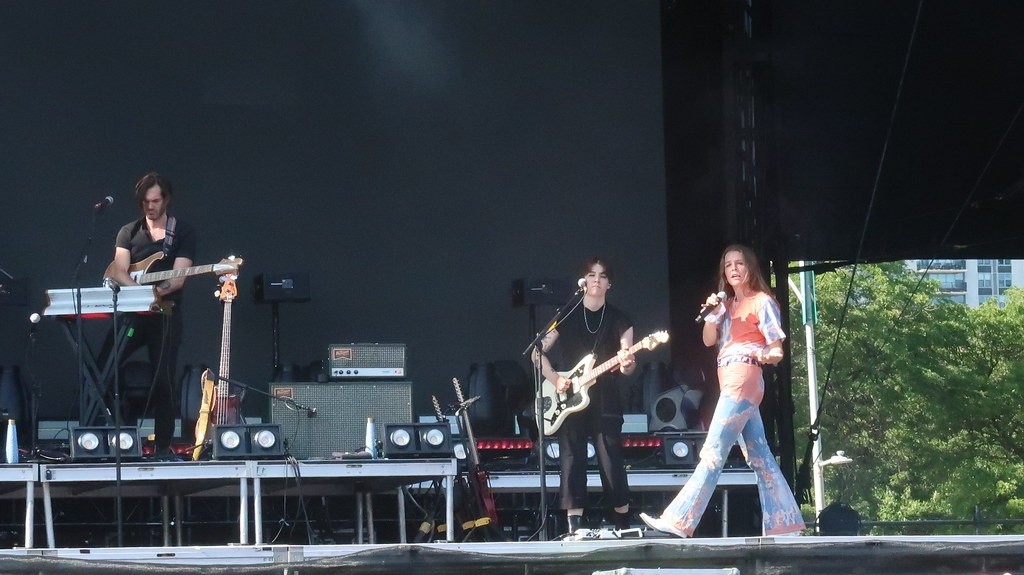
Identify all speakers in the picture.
[268,380,414,460]
[0,363,32,463]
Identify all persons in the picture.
[83,171,196,461]
[530,255,637,538]
[639,244,808,541]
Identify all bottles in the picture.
[365,417,378,459]
[6,419,20,464]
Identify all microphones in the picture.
[578,278,587,293]
[95,196,114,208]
[695,291,727,323]
[308,407,317,418]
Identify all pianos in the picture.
[41,284,173,427]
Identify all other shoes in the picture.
[639,512,688,539]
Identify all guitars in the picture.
[431,377,498,532]
[534,329,670,436]
[194,255,247,443]
[103,250,245,291]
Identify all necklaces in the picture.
[583,297,606,334]
